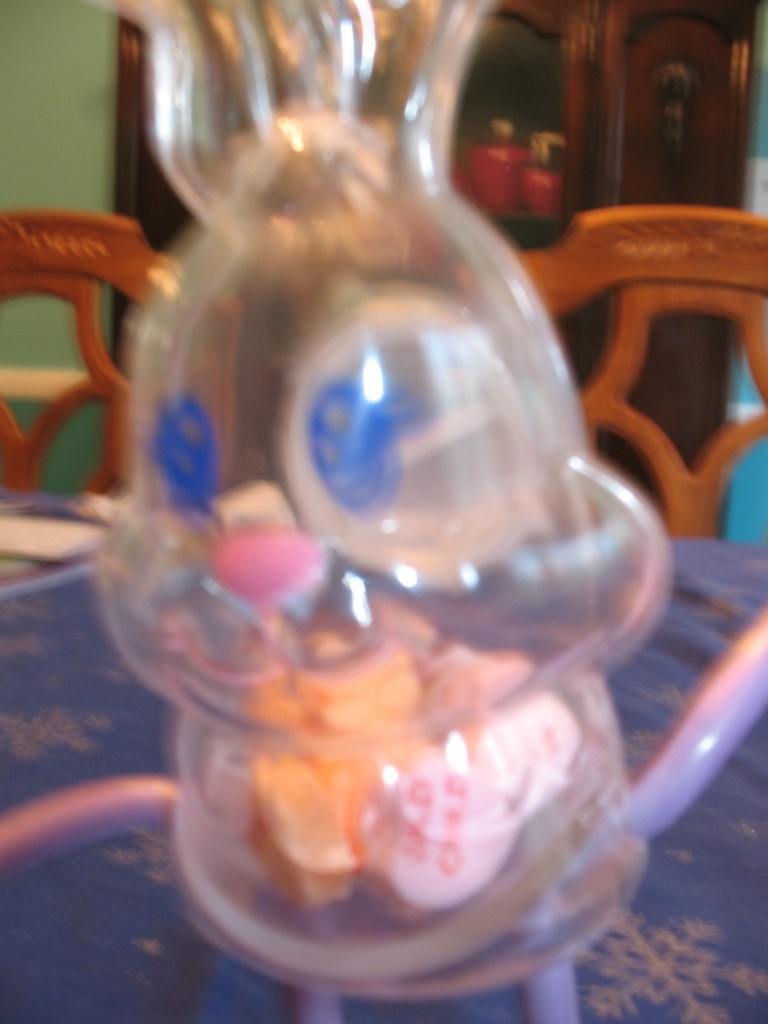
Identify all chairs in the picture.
[0,209,244,501]
[520,202,768,537]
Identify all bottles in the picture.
[524,132,563,212]
[471,118,533,210]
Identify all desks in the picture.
[0,490,768,1024]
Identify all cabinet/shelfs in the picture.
[110,0,760,514]
